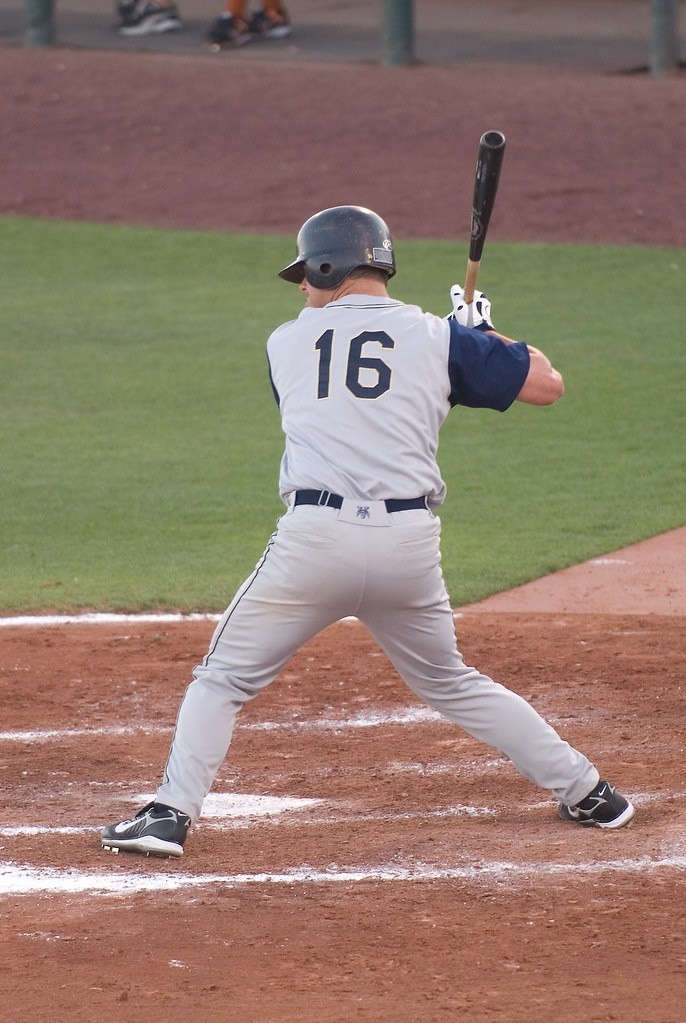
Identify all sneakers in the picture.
[205,15,254,48]
[101,800,190,859]
[558,781,635,829]
[120,4,182,34]
[251,8,291,36]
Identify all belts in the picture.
[295,489,428,513]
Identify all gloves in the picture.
[444,285,496,332]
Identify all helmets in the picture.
[278,204,396,289]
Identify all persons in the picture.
[115,0,182,38]
[205,0,293,54]
[100,203,634,857]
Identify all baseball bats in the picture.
[464,130,507,304]
